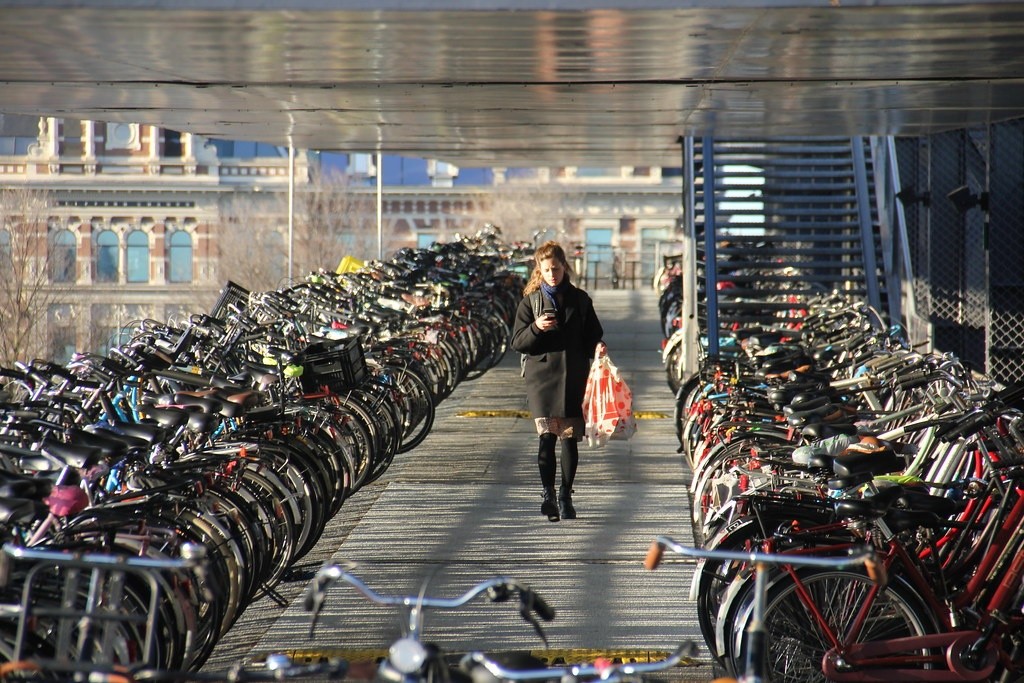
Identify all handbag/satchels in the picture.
[583,349,636,448]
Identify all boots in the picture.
[558,486,576,519]
[540,487,561,522]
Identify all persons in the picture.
[510,240,608,522]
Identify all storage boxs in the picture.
[286,335,369,395]
[210,280,250,319]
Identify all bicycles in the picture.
[460,636,703,682]
[302,551,561,683]
[645,234,1024,682]
[0,221,547,682]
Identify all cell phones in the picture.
[543,307,556,321]
